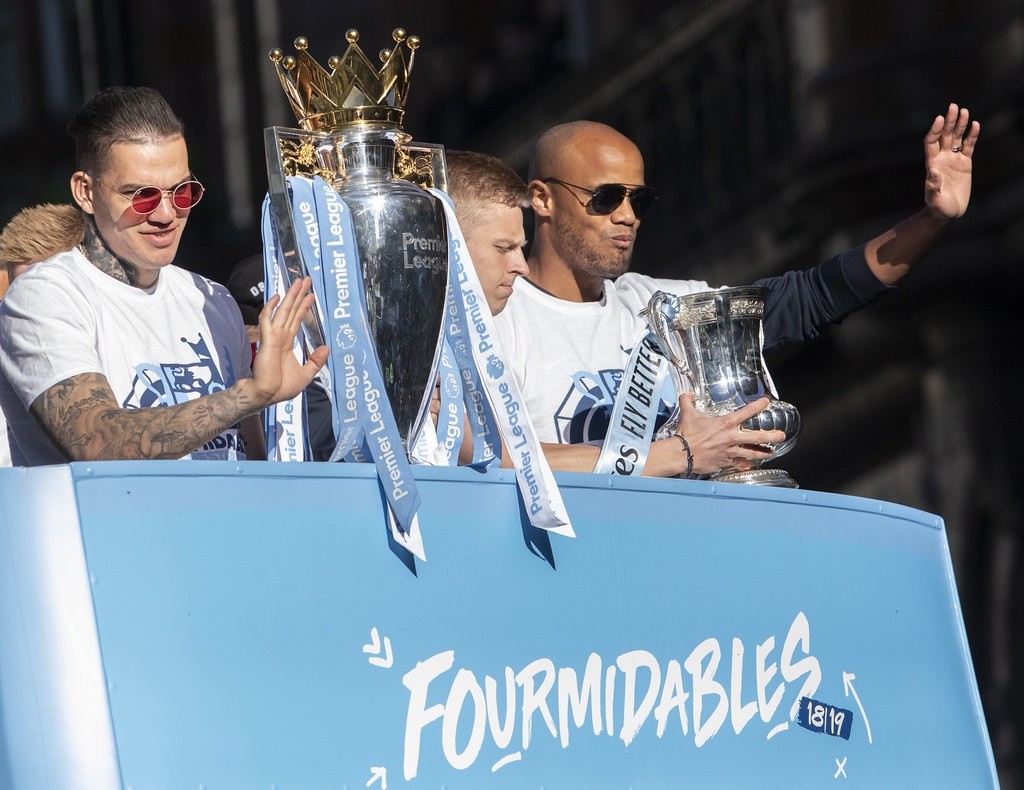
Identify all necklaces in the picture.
[78,243,86,258]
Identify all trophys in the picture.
[648,283,801,488]
[272,30,448,464]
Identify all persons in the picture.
[0,86,440,467]
[0,202,82,285]
[459,104,980,474]
[441,152,529,316]
[232,255,264,343]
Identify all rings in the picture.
[952,146,962,151]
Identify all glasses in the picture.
[542,176,660,221]
[87,172,207,215]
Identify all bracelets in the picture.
[674,435,693,476]
